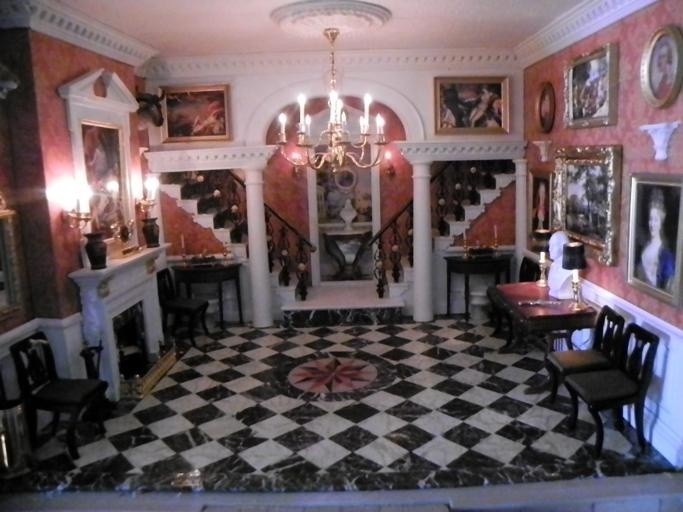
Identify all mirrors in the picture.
[57,66,142,269]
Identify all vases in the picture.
[141,217,159,248]
[84,232,107,268]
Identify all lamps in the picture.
[269,1,393,174]
[533,229,550,288]
[562,241,588,311]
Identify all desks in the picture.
[486,283,596,355]
[444,255,513,320]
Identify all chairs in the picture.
[491,256,540,316]
[172,261,246,333]
[156,268,210,348]
[9,332,108,460]
[544,306,624,410]
[564,323,660,459]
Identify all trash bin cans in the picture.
[1,398,35,484]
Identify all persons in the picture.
[637,187,675,295]
[532,181,548,229]
[655,41,673,99]
[547,231,573,299]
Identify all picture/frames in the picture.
[157,85,230,143]
[639,25,682,108]
[627,173,683,307]
[552,145,621,266]
[525,161,553,254]
[533,80,555,134]
[560,43,617,128]
[433,77,511,134]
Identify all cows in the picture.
[132,83,166,127]
